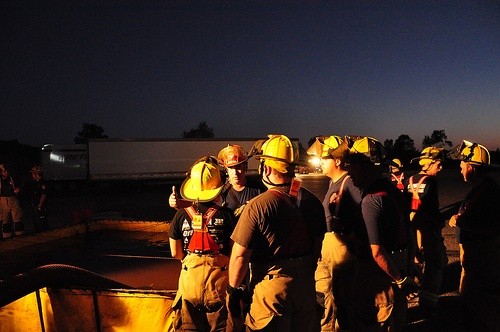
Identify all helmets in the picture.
[179,163,227,202]
[259,134,298,173]
[457,140,491,164]
[320,134,348,159]
[418,146,445,165]
[352,136,383,157]
[31,166,43,173]
[390,159,402,167]
[219,144,250,168]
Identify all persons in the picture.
[163,135,500,332]
[0,164,49,240]
